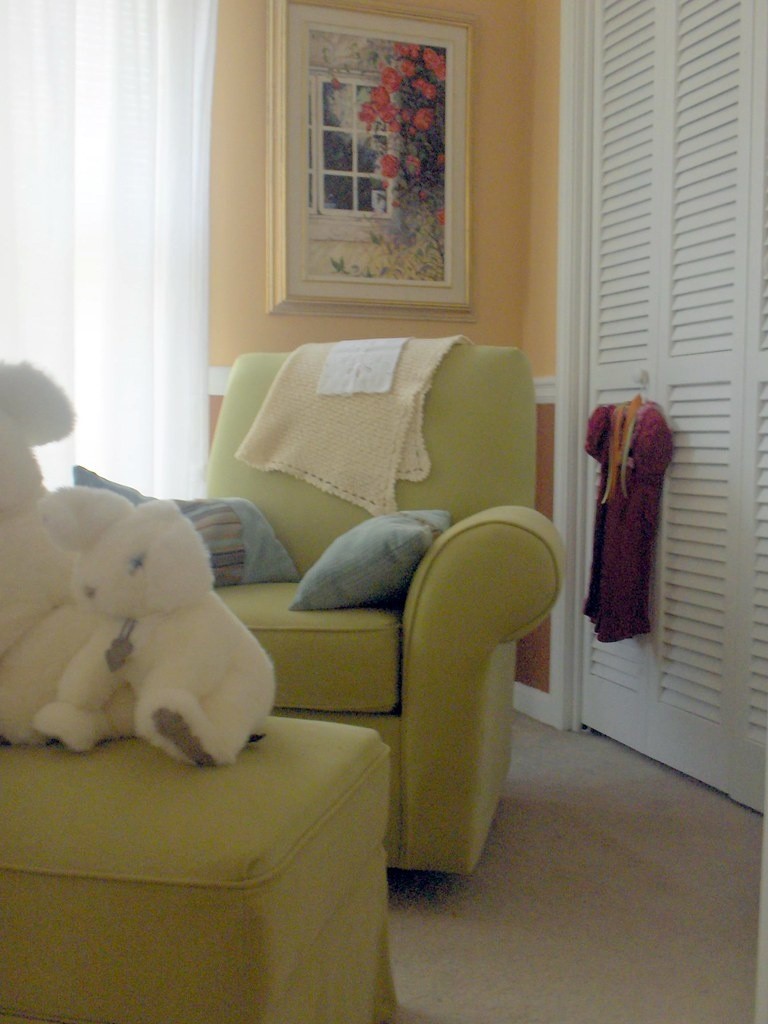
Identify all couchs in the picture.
[211,345,566,892]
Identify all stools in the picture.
[1,712,398,1024]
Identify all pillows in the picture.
[73,467,301,584]
[288,509,453,611]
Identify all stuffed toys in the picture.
[0,357,278,768]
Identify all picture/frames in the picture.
[264,2,477,320]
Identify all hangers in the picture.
[617,376,647,408]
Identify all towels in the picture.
[580,401,675,644]
[234,334,475,521]
